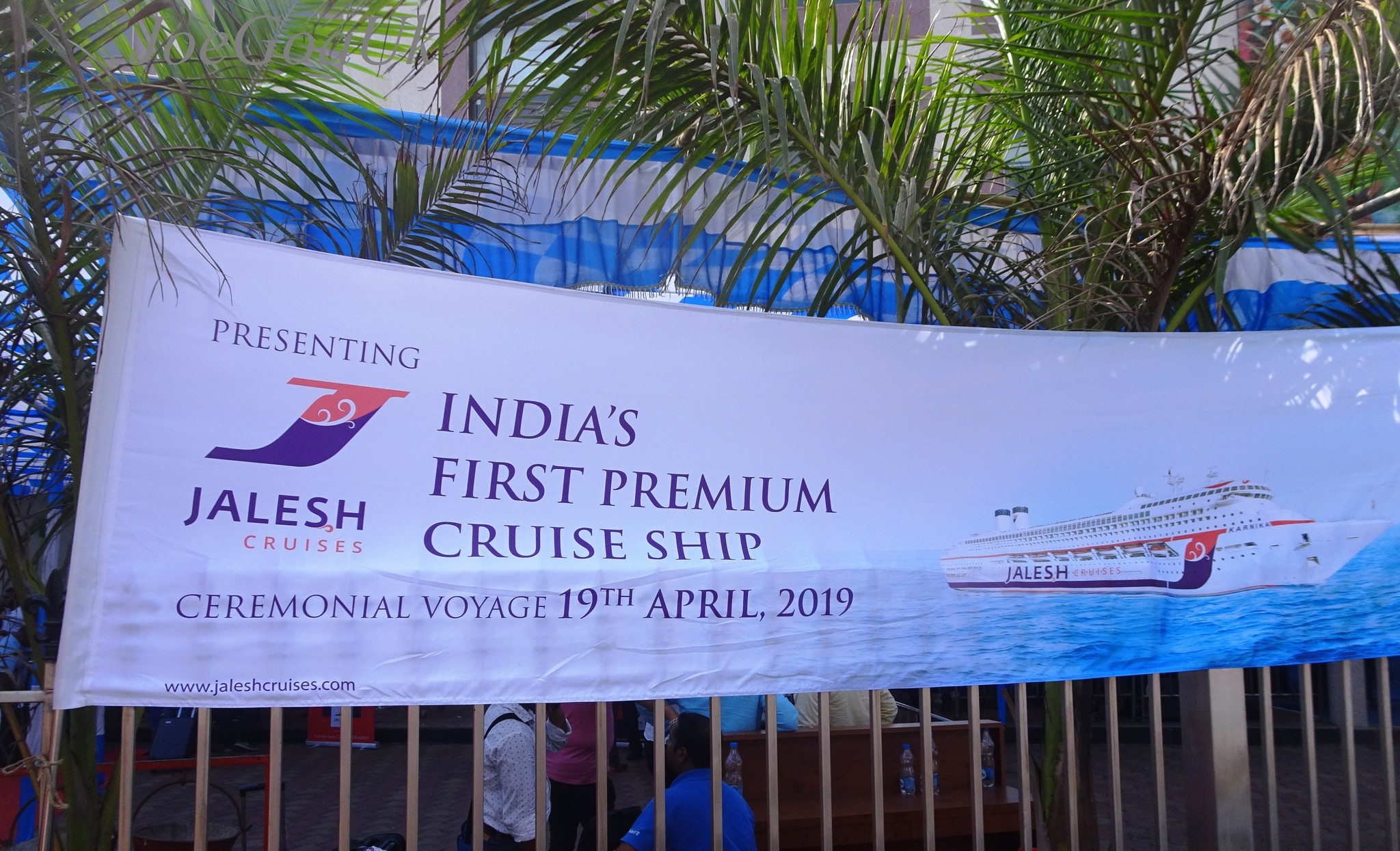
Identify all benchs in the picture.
[721,719,1037,851]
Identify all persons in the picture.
[224,709,258,753]
[616,712,758,851]
[637,694,799,786]
[794,688,898,728]
[150,707,191,739]
[483,702,572,851]
[546,698,681,851]
[609,703,627,773]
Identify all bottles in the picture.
[920,732,940,795]
[900,744,916,796]
[725,742,743,797]
[980,728,996,788]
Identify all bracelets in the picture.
[669,718,678,729]
